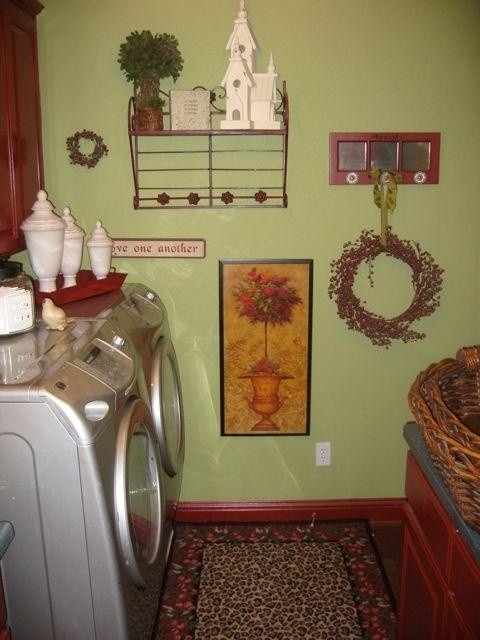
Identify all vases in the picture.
[237,373,295,431]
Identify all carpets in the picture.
[150,513,401,639]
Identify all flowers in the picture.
[232,268,303,374]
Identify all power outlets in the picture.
[314,442,332,468]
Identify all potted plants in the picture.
[117,30,185,104]
[133,94,167,134]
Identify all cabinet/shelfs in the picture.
[400,446,480,640]
[1,1,50,262]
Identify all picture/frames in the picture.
[217,257,313,438]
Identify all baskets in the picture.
[406,346,480,529]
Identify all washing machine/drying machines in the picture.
[0,282,186,639]
